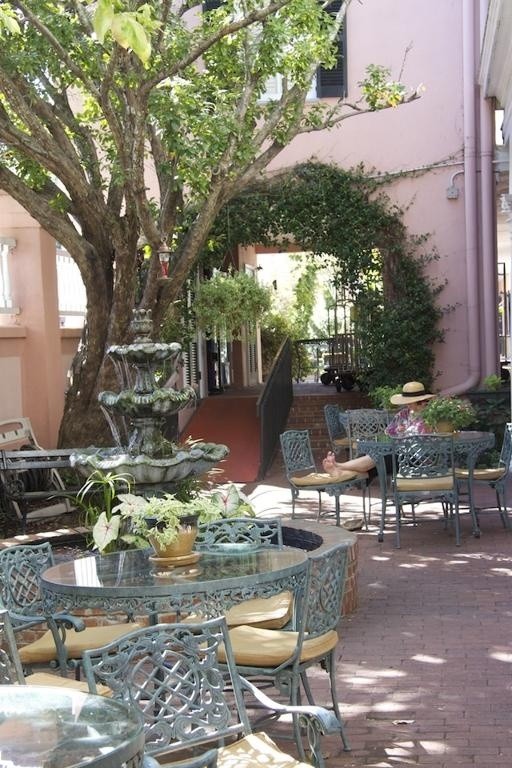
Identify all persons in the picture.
[322,381,438,490]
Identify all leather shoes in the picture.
[342,519,365,530]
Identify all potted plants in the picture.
[120,494,222,567]
[412,394,480,434]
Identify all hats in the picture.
[390,380,438,405]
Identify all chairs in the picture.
[1,610,112,698]
[444,422,511,534]
[387,432,461,550]
[345,408,405,520]
[196,517,284,545]
[279,430,369,532]
[322,403,358,457]
[190,544,354,768]
[1,540,146,681]
[82,616,314,768]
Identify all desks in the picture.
[39,544,308,727]
[356,429,497,539]
[0,676,146,768]
